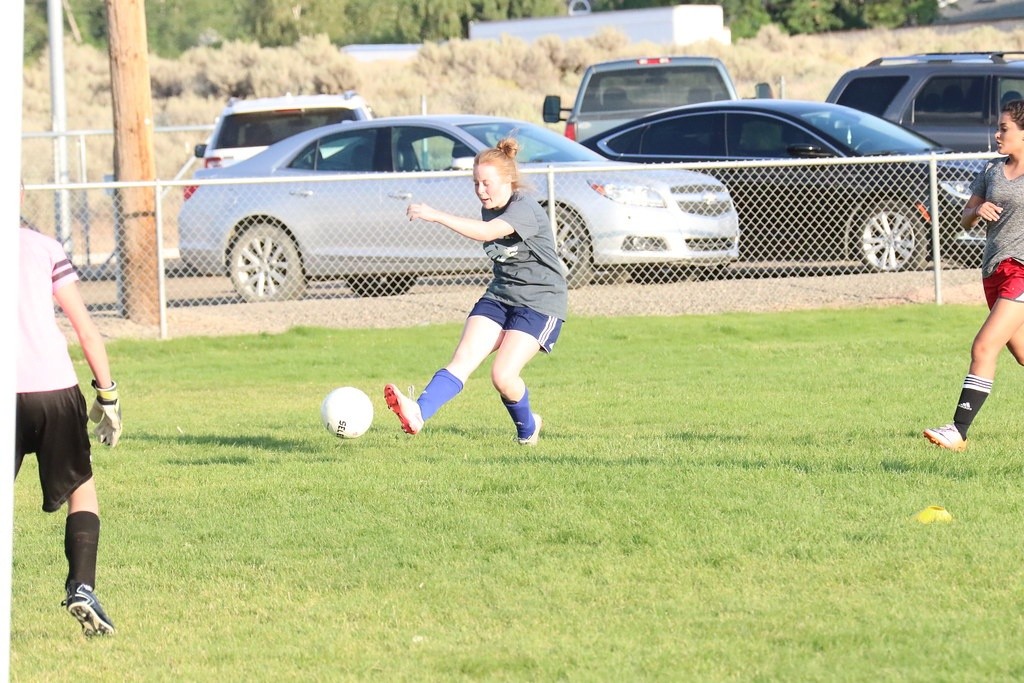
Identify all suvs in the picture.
[817,49,1024,170]
[195,91,379,174]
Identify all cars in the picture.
[541,57,775,145]
[177,114,742,306]
[526,99,998,274]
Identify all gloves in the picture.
[87,380,122,448]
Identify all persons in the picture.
[383,138,566,448]
[14,185,122,642]
[923,100,1024,452]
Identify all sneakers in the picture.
[384,384,424,435]
[62,582,115,639]
[512,413,542,445]
[922,425,967,453]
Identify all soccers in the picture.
[321,387,374,440]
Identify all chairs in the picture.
[245,113,352,145]
[1001,90,1022,106]
[782,127,811,154]
[708,116,744,156]
[602,88,713,108]
[923,94,938,113]
[943,85,964,107]
[397,138,419,170]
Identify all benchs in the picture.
[299,145,373,168]
